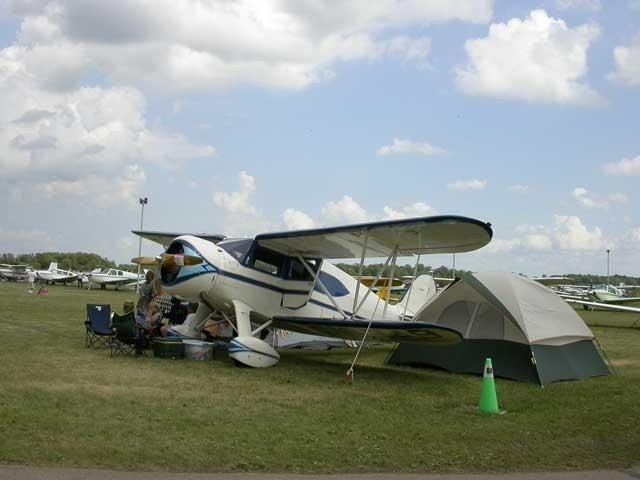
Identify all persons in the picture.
[27,267,40,294]
[75,269,85,288]
[35,271,41,286]
[132,270,236,349]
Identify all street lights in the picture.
[136,197,147,294]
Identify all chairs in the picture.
[111,309,148,357]
[85,303,116,350]
[137,312,164,349]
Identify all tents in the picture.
[383,267,617,390]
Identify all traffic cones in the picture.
[476,358,499,416]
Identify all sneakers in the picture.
[27,289,33,294]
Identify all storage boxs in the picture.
[153,340,183,359]
[181,339,215,361]
